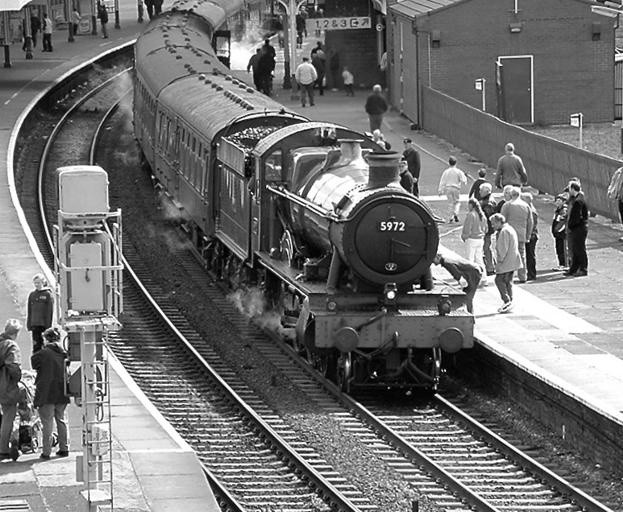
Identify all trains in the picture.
[131,0,475,400]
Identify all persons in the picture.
[402,139,420,198]
[27,274,55,353]
[0,319,23,458]
[365,84,388,132]
[462,143,589,313]
[342,66,355,96]
[438,156,467,223]
[22,0,163,52]
[31,328,71,459]
[247,6,339,107]
[399,161,413,193]
[607,166,623,241]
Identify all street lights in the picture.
[476,78,487,112]
[570,113,583,148]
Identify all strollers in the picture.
[7,369,59,454]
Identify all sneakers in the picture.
[448,216,459,224]
[552,265,587,276]
[57,451,68,456]
[40,453,50,460]
[0,439,22,462]
[498,301,513,313]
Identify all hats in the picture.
[404,138,412,143]
